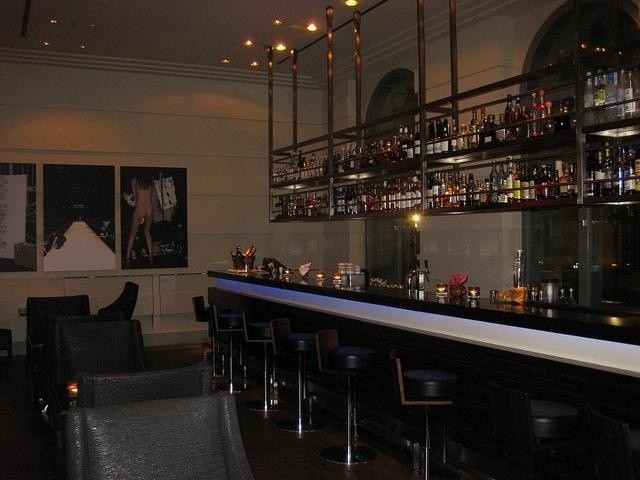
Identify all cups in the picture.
[312,270,342,285]
[434,283,481,301]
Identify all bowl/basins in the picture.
[233,255,256,271]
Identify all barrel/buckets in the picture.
[233,255,254,269]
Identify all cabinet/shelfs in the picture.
[265,49,640,222]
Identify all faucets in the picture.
[408,269,431,292]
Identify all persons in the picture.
[122,167,158,265]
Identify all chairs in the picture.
[316,330,379,464]
[189,288,318,439]
[0,279,213,422]
[498,386,581,479]
[64,390,257,480]
[387,350,463,480]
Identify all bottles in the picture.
[528,286,576,305]
[582,66,637,126]
[584,144,640,199]
